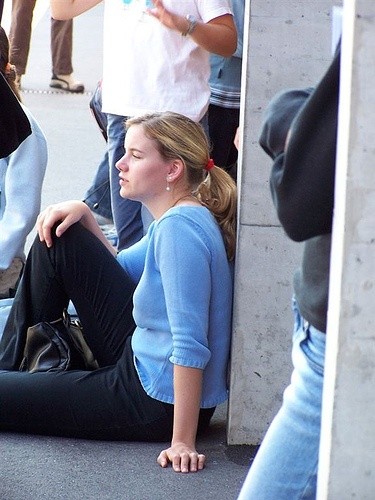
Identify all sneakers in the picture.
[49,73,84,92]
[15,73,21,90]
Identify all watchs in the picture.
[180,14,198,37]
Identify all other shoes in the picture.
[92,212,117,246]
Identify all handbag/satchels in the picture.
[89,81,107,142]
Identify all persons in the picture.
[236,35,341,500]
[0,110,238,472]
[0,0,246,299]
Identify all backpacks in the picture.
[19,308,100,372]
